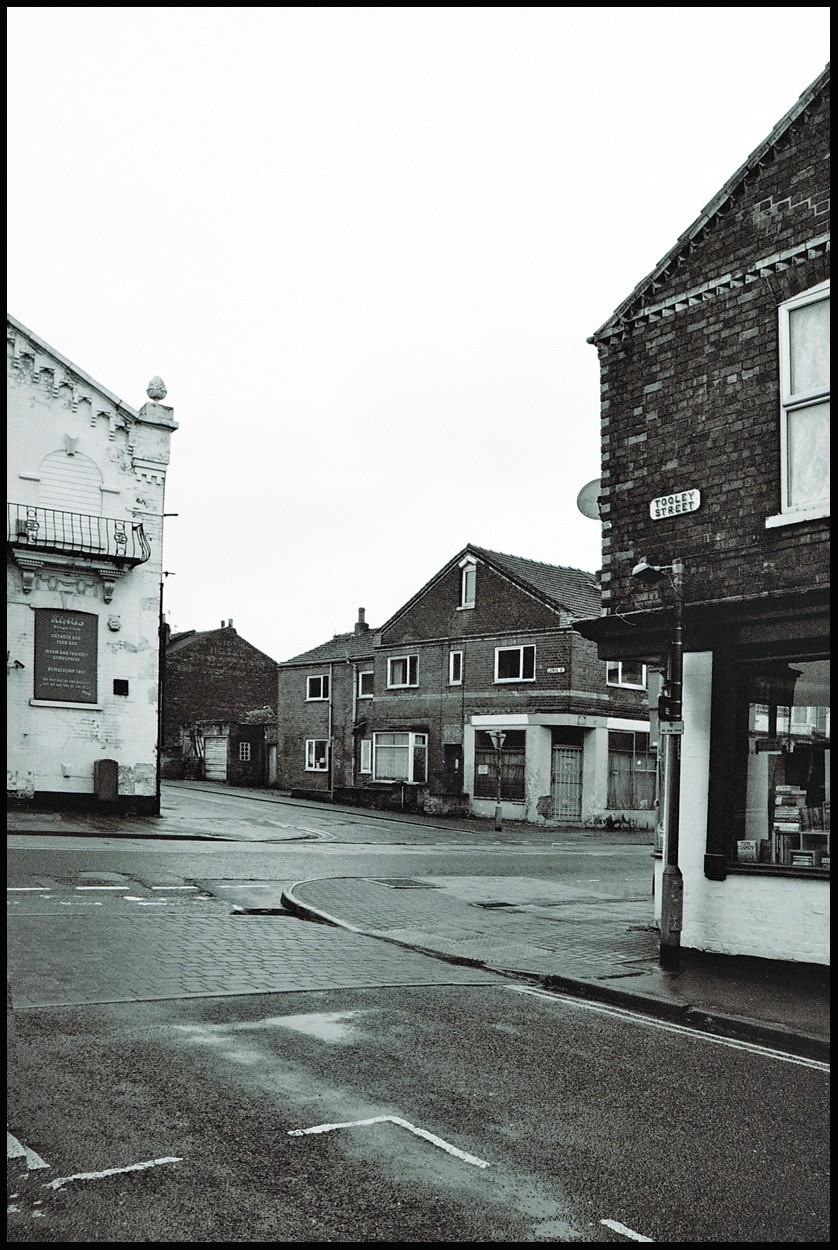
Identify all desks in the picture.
[770,830,830,869]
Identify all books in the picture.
[736,783,831,870]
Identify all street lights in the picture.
[630,562,684,971]
